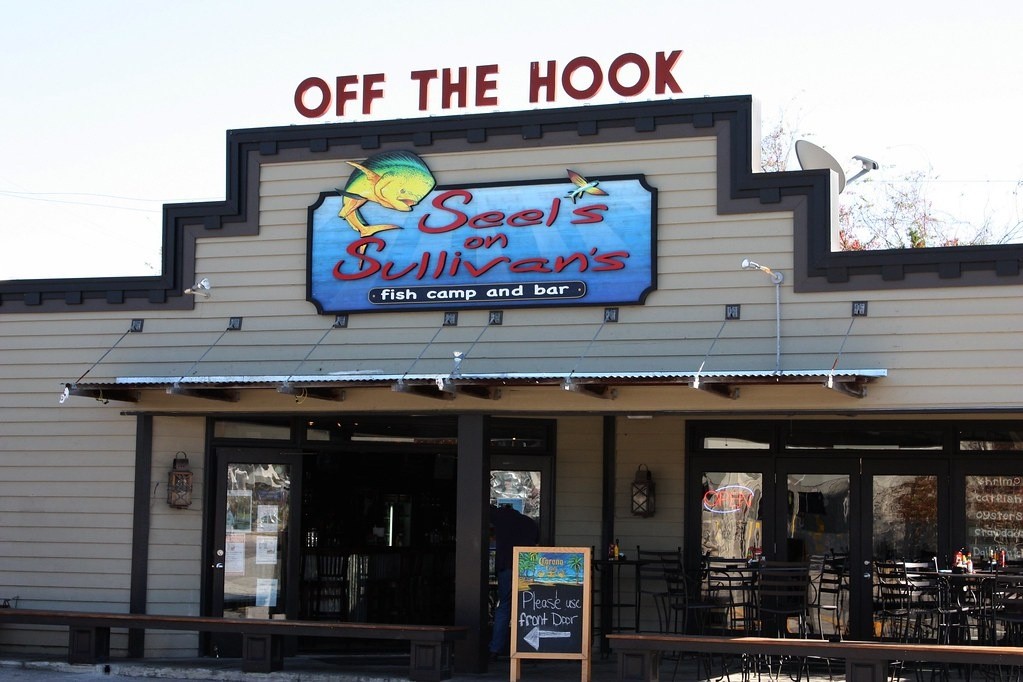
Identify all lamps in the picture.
[631,465,656,518]
[167,452,193,509]
[184,278,211,300]
[741,257,784,284]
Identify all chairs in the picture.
[874,554,1023,682]
[830,548,851,637]
[634,545,847,682]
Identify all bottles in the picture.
[614,543,618,560]
[616,539,620,552]
[955,551,962,567]
[967,553,972,561]
[962,553,967,565]
[999,550,1005,568]
[758,556,766,568]
[967,561,973,572]
[992,559,997,570]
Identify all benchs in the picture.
[605,632,1023,682]
[0,607,472,682]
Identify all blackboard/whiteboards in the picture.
[510,546,591,659]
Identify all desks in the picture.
[890,571,1023,614]
[708,567,809,603]
[591,560,639,661]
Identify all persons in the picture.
[950,545,976,608]
[993,546,1008,572]
[488,504,540,663]
